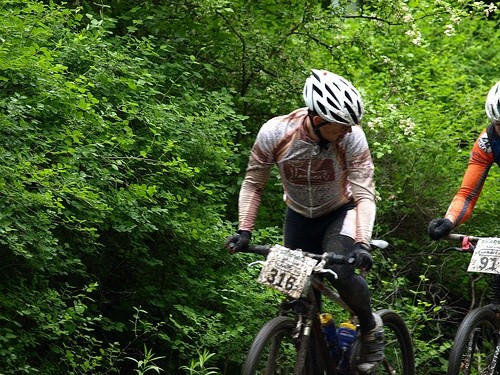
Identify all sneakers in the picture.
[356,312,385,374]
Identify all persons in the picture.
[227,68,385,375]
[427,81,500,242]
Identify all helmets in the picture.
[302,69,364,125]
[485,79,500,122]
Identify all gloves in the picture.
[343,242,374,273]
[427,218,453,240]
[226,230,252,255]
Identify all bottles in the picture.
[319,314,357,367]
